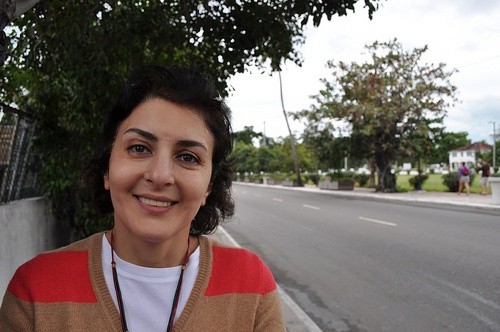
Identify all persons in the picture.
[457,162,470,195]
[477,159,491,196]
[0,66,283,332]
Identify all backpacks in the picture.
[461,166,468,176]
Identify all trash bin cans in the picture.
[487,177,500,205]
